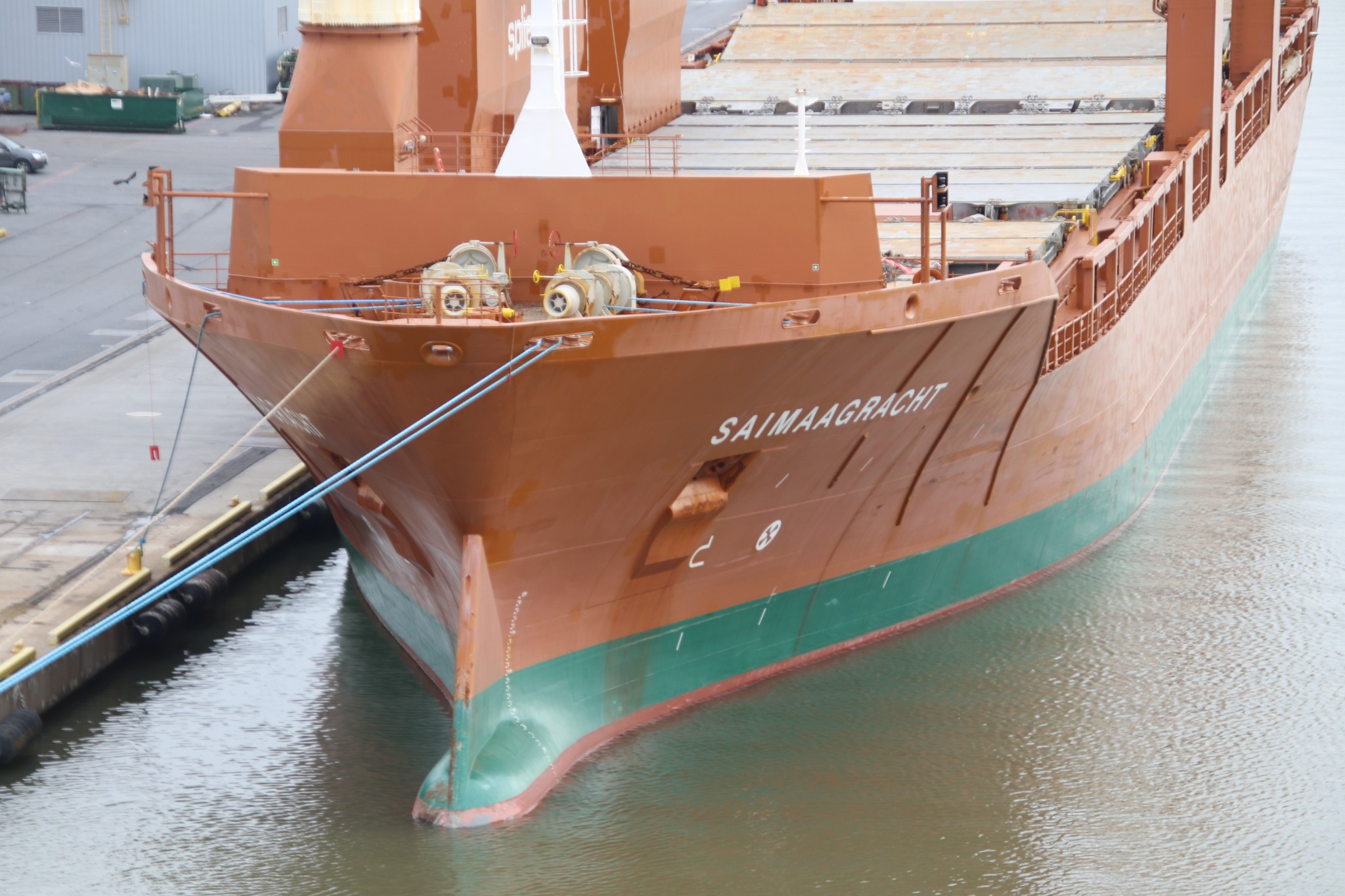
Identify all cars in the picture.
[0,134,47,175]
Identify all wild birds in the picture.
[113,171,137,185]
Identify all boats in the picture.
[127,0,1325,839]
